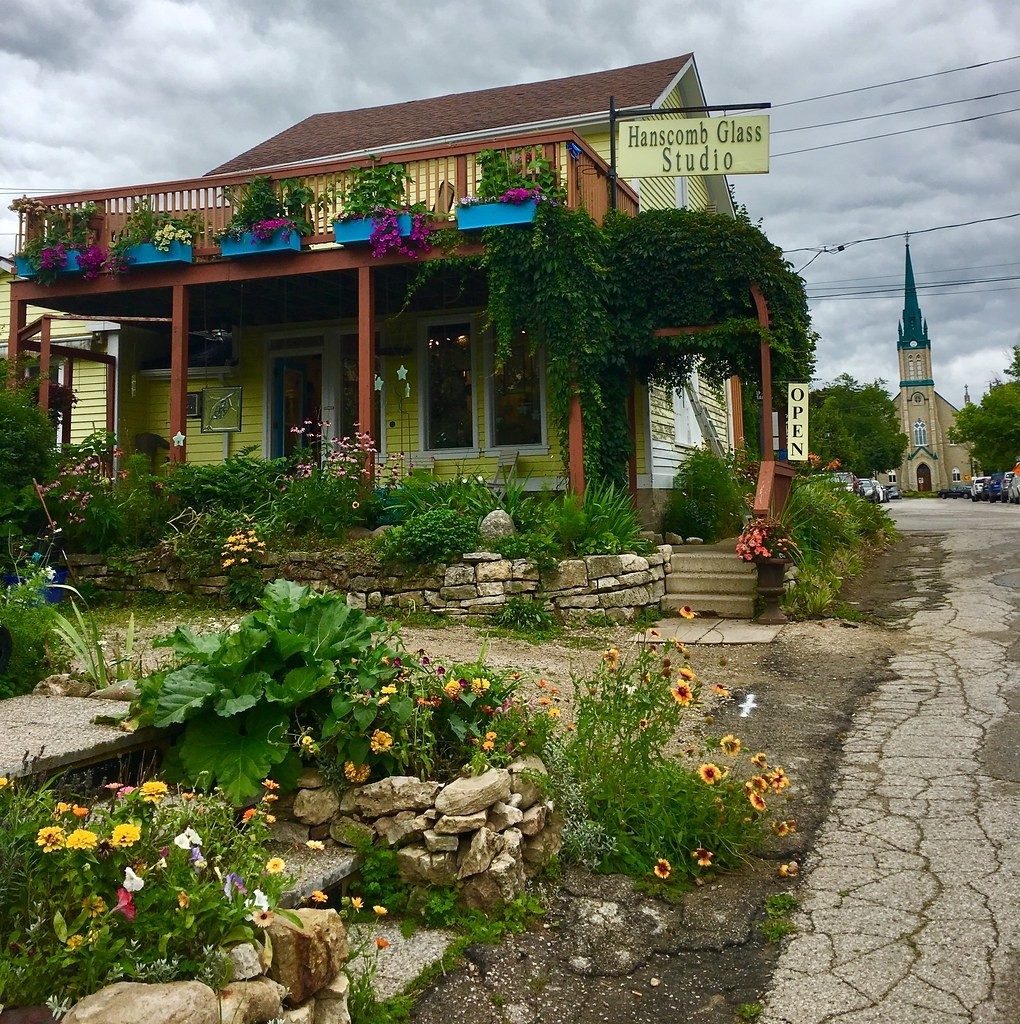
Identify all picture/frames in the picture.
[185,391,200,418]
[199,386,242,432]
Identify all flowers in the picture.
[329,153,456,262]
[454,143,568,207]
[99,196,197,281]
[8,232,111,286]
[9,196,48,227]
[211,166,313,244]
[736,480,828,574]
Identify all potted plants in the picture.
[82,201,105,231]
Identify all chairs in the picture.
[405,452,435,489]
[486,450,520,506]
[428,180,455,213]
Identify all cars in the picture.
[1000,471,1013,500]
[826,473,863,495]
[979,478,990,501]
[872,480,889,503]
[1009,463,1020,504]
[859,478,879,504]
[989,473,1003,502]
[886,486,903,499]
[937,485,972,499]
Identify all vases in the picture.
[118,240,193,272]
[457,199,538,233]
[220,228,301,259]
[334,212,414,246]
[747,558,793,625]
[14,248,89,278]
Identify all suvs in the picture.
[971,477,991,502]
